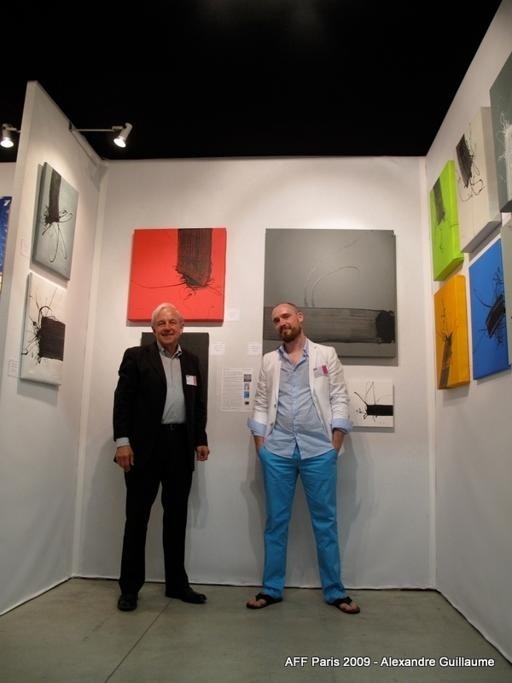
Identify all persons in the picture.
[112,301,210,612]
[246,303,361,613]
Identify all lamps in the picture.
[69,123,136,149]
[0,123,20,148]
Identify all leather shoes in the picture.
[165,587,207,601]
[117,591,138,611]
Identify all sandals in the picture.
[331,595,360,614]
[246,593,283,609]
